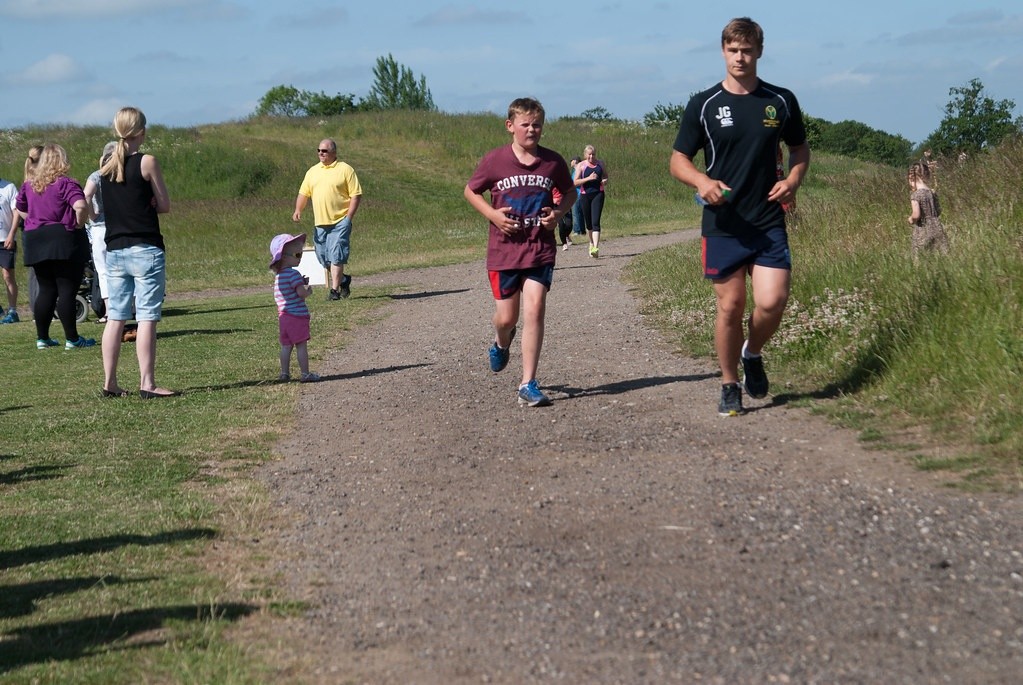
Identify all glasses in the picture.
[317,149,328,153]
[283,252,303,259]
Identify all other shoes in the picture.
[280,373,291,382]
[300,372,320,382]
[139,390,181,400]
[563,244,568,251]
[567,237,573,246]
[102,388,137,398]
[589,246,599,257]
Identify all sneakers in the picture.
[718,382,745,415]
[339,273,351,297]
[37,338,59,349]
[65,336,96,350]
[329,289,340,300]
[740,339,768,399]
[518,379,550,406]
[489,326,516,372]
[0,312,19,324]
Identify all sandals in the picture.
[94,315,108,323]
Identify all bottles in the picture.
[694,189,730,205]
[777,171,796,217]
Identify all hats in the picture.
[269,233,306,267]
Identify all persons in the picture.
[670,18,810,418]
[906,147,950,263]
[0,141,123,350]
[464,97,579,406]
[268,233,321,381]
[557,145,608,258]
[293,140,362,300]
[81,107,181,398]
